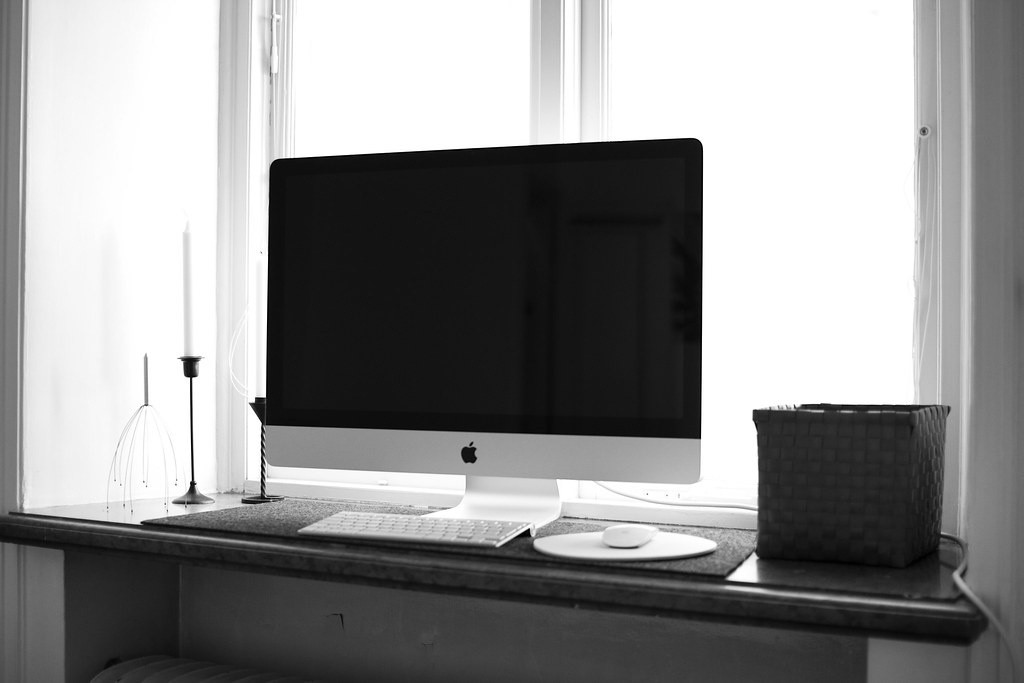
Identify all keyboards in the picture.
[297,510,535,548]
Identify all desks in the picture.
[0,493,988,683]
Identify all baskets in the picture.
[754,401,951,566]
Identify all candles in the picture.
[184,219,198,356]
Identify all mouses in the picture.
[601,525,659,547]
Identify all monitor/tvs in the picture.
[266,136,704,528]
